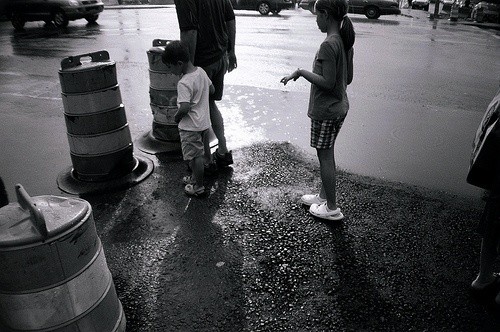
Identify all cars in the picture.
[0,0,105,30]
[298,0,402,19]
[229,0,293,16]
[410,0,500,24]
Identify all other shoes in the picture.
[212,148,233,165]
[189,160,218,170]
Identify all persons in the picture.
[173,0,238,167]
[162,41,216,196]
[280,0,356,220]
[466,96,500,304]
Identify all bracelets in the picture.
[298,69,302,76]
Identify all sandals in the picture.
[184,184,206,196]
[302,194,326,206]
[309,201,344,220]
[183,175,196,185]
